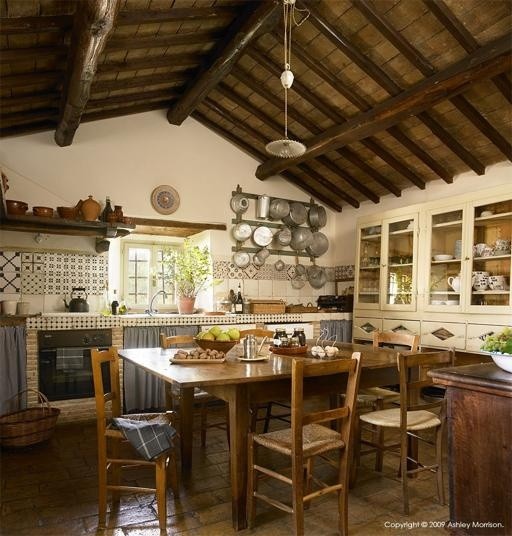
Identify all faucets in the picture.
[144,290,168,316]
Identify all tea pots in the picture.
[448,271,477,292]
[63,288,89,312]
[237,333,268,359]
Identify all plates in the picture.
[169,356,227,364]
[434,254,453,261]
[237,357,268,362]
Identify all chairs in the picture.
[247,352,363,535]
[239,328,292,433]
[90,347,180,531]
[359,345,456,518]
[340,332,422,478]
[159,332,232,451]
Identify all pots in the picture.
[250,226,273,247]
[305,231,329,257]
[6,200,28,215]
[282,202,308,227]
[309,206,327,229]
[290,228,314,250]
[233,222,253,242]
[32,206,53,217]
[269,199,290,219]
[233,251,250,268]
[274,230,292,246]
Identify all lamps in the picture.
[265,2,308,158]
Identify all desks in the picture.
[426,362,511,536]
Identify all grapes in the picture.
[480,326,512,354]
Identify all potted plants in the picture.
[479,327,512,375]
[152,239,225,316]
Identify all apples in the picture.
[227,328,240,340]
[216,333,230,341]
[202,333,214,340]
[198,331,204,339]
[210,326,221,335]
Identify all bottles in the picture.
[244,302,251,314]
[290,338,299,347]
[281,338,288,347]
[234,286,243,313]
[274,328,287,347]
[111,290,119,315]
[292,328,306,347]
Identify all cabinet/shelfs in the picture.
[424,196,511,317]
[353,216,417,312]
[25,313,354,425]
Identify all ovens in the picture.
[37,329,113,404]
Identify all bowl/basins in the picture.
[193,336,240,356]
[480,349,512,373]
[471,271,489,291]
[230,193,249,215]
[431,300,445,305]
[57,207,80,220]
[445,301,459,305]
[487,275,504,290]
